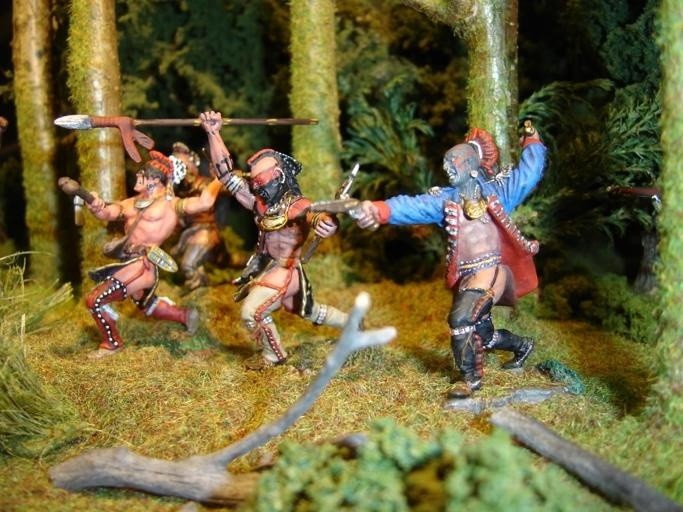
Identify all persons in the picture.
[356,124,546,400]
